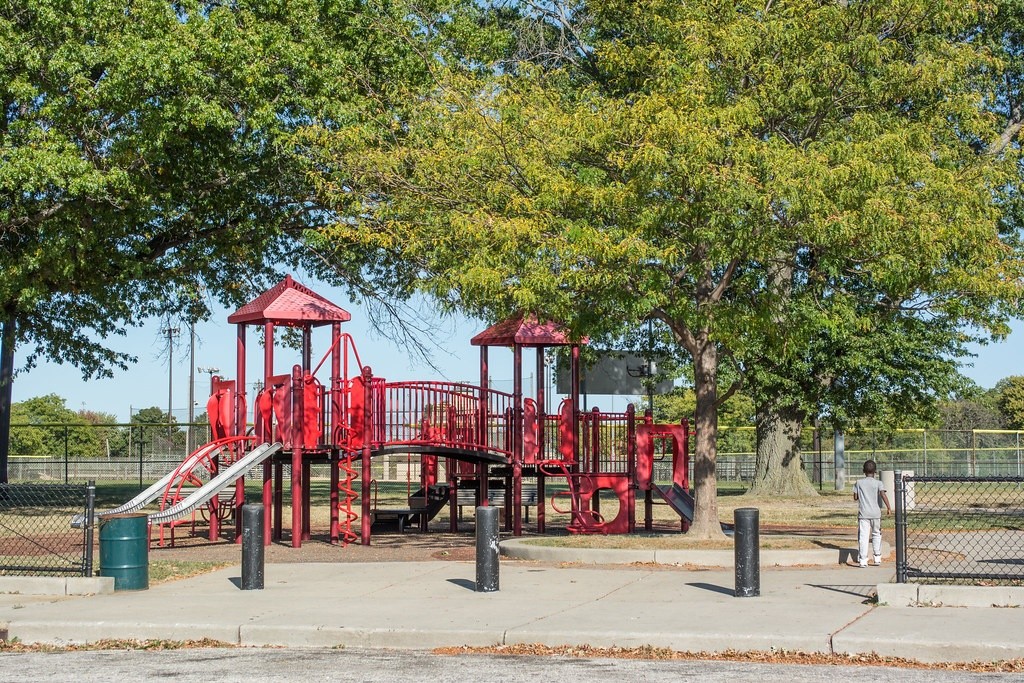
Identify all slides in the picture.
[70,442,236,530]
[146,441,284,525]
[650,481,737,536]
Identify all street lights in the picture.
[162,328,181,442]
[197,368,219,378]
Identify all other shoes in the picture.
[874,562,880,565]
[860,564,869,568]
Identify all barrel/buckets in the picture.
[99,513,149,591]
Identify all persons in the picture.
[854,460,891,567]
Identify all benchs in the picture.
[157,487,236,526]
[446,484,538,524]
